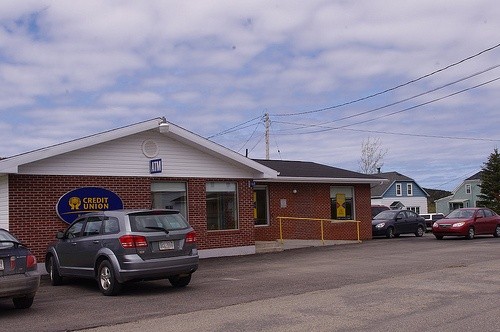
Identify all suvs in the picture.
[45,208,200,297]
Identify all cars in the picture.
[371,209,427,239]
[0,227,39,310]
[418,213,446,227]
[432,207,500,239]
